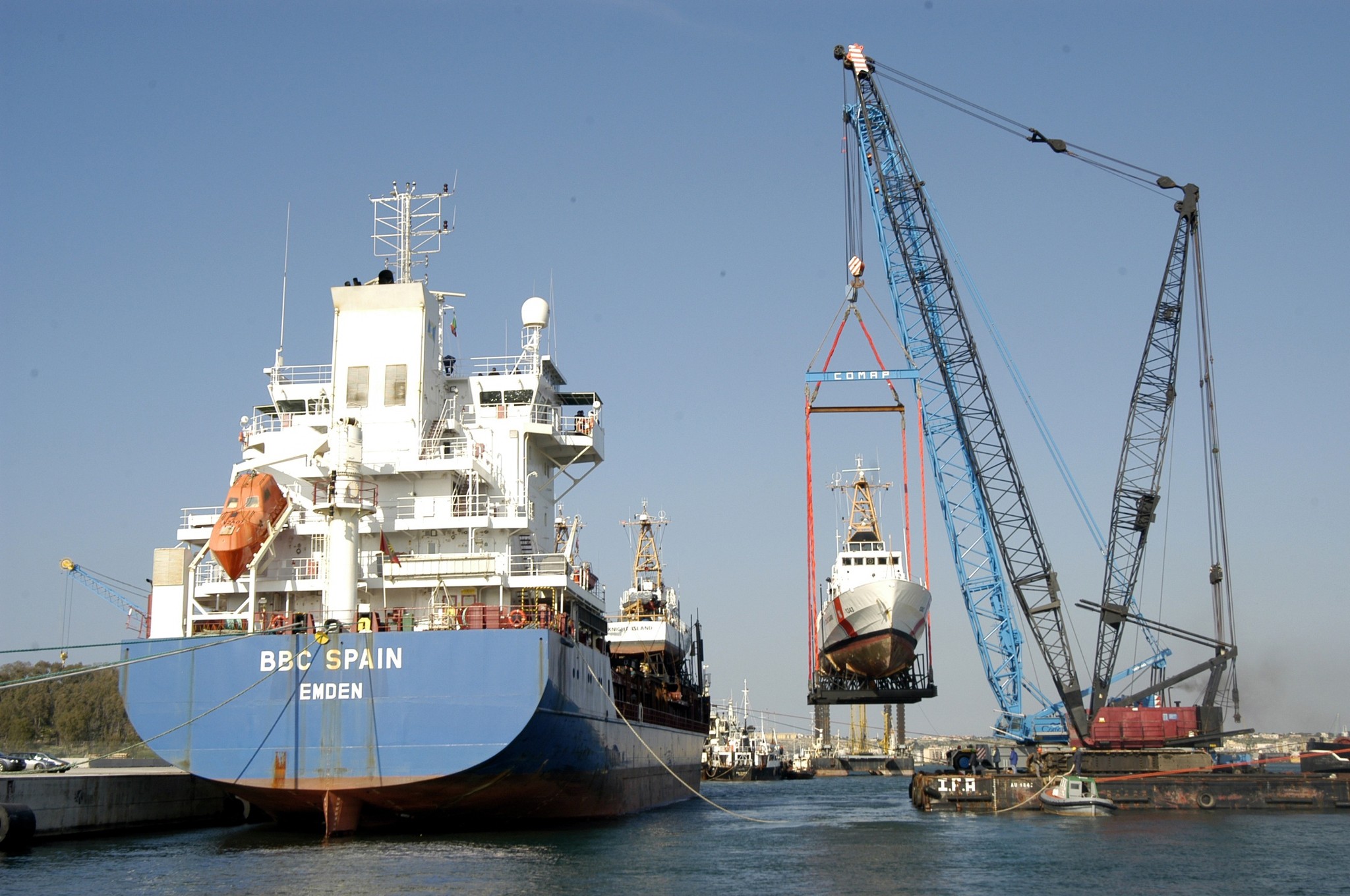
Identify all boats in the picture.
[604,495,691,668]
[817,447,932,679]
[1037,774,1118,818]
[208,468,288,582]
[118,168,711,838]
[700,678,817,780]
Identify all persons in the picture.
[992,745,1000,774]
[615,665,636,678]
[477,373,483,376]
[968,749,977,775]
[1072,747,1082,776]
[509,365,522,374]
[488,367,499,375]
[1033,748,1042,778]
[1009,748,1018,774]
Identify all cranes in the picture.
[830,42,1257,751]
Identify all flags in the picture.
[450,316,457,337]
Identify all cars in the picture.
[0,752,57,773]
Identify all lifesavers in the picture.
[1197,791,1216,808]
[508,609,525,627]
[576,418,592,433]
[272,615,288,633]
[725,747,729,752]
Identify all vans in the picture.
[9,751,71,774]
